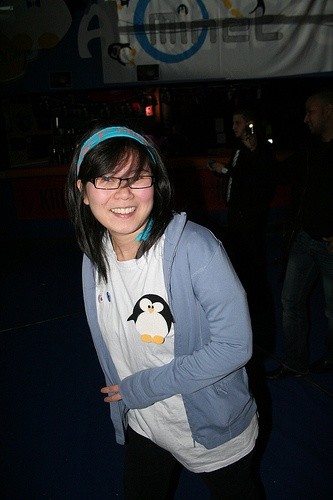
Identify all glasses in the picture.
[87,176,154,190]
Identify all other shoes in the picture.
[310,359,332,373]
[265,365,296,381]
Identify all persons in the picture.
[205,109,282,352]
[266,90,333,379]
[65,123,261,500]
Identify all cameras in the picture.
[208,160,216,169]
